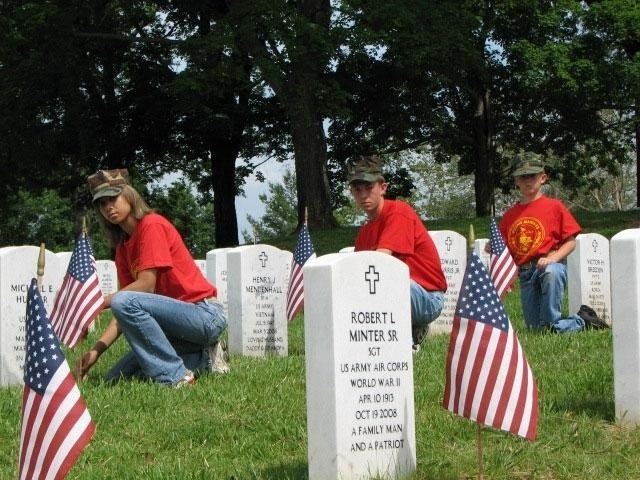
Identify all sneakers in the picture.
[411,323,430,350]
[174,370,197,388]
[578,303,610,329]
[204,340,231,376]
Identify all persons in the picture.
[482,151,610,334]
[348,161,448,354]
[75,168,227,389]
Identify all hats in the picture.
[347,156,380,185]
[511,150,545,177]
[87,167,130,203]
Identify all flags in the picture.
[441,251,539,442]
[17,278,95,480]
[48,231,106,352]
[286,223,317,323]
[488,217,519,298]
